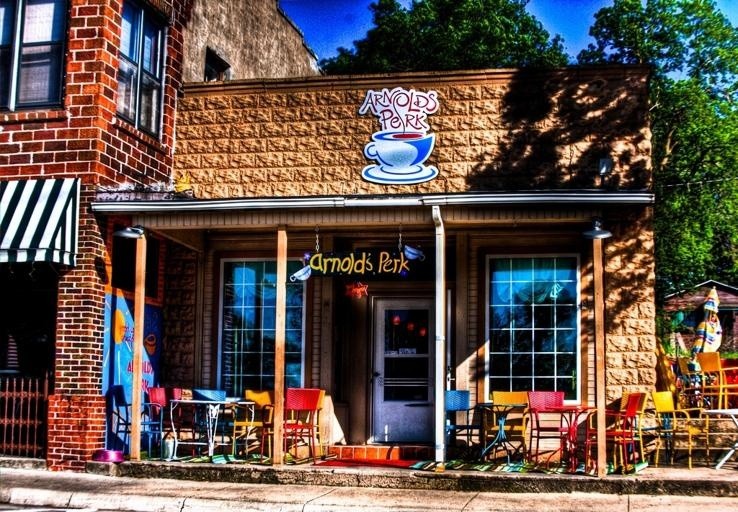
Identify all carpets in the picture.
[306,455,430,470]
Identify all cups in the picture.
[143,333,157,356]
[364,130,436,169]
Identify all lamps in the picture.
[580,213,613,240]
[111,225,146,239]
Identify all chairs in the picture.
[656,347,738,476]
[443,386,656,476]
[110,382,327,467]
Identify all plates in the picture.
[361,164,441,183]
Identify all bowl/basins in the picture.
[90,448,125,463]
